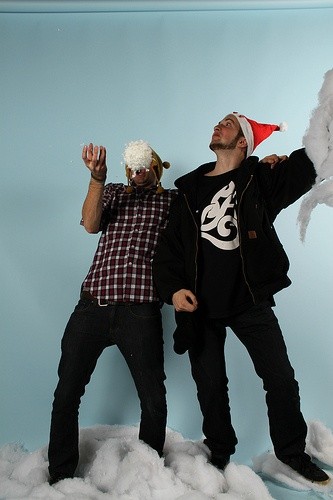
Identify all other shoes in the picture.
[294,461,331,485]
[211,454,231,471]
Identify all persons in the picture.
[47,139,289,488]
[152,111,333,485]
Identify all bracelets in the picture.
[91,172,107,182]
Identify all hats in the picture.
[231,111,287,160]
[125,146,170,194]
